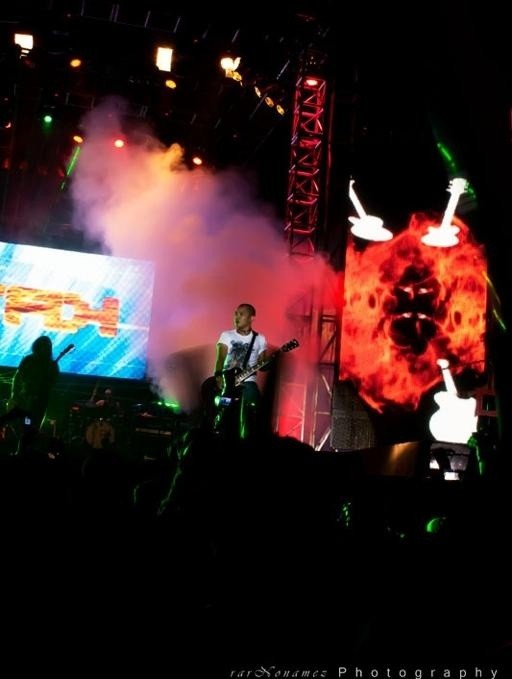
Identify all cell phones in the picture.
[24,416,33,430]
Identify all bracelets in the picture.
[214,371,224,377]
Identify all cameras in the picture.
[219,396,233,407]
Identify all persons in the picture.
[6,334,60,437]
[213,301,283,444]
[2,430,512,679]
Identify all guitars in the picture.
[200,338,299,411]
